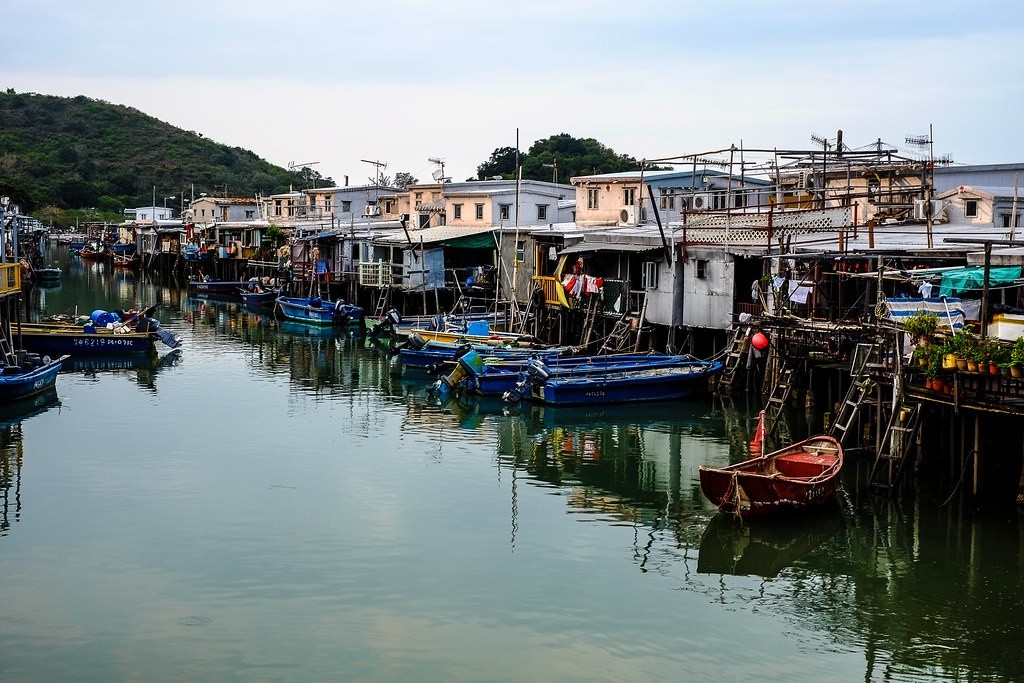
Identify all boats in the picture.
[55,235,138,257]
[276,295,362,326]
[697,432,847,519]
[240,289,288,305]
[0,358,63,402]
[188,277,261,292]
[37,267,62,281]
[362,309,724,405]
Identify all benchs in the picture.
[776,456,834,478]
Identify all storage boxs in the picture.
[987,314,1024,342]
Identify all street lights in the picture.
[164,197,175,219]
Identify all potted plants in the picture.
[902,313,1024,399]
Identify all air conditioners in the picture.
[619,205,641,226]
[364,206,379,214]
[693,193,714,211]
[914,200,942,219]
[799,171,814,189]
